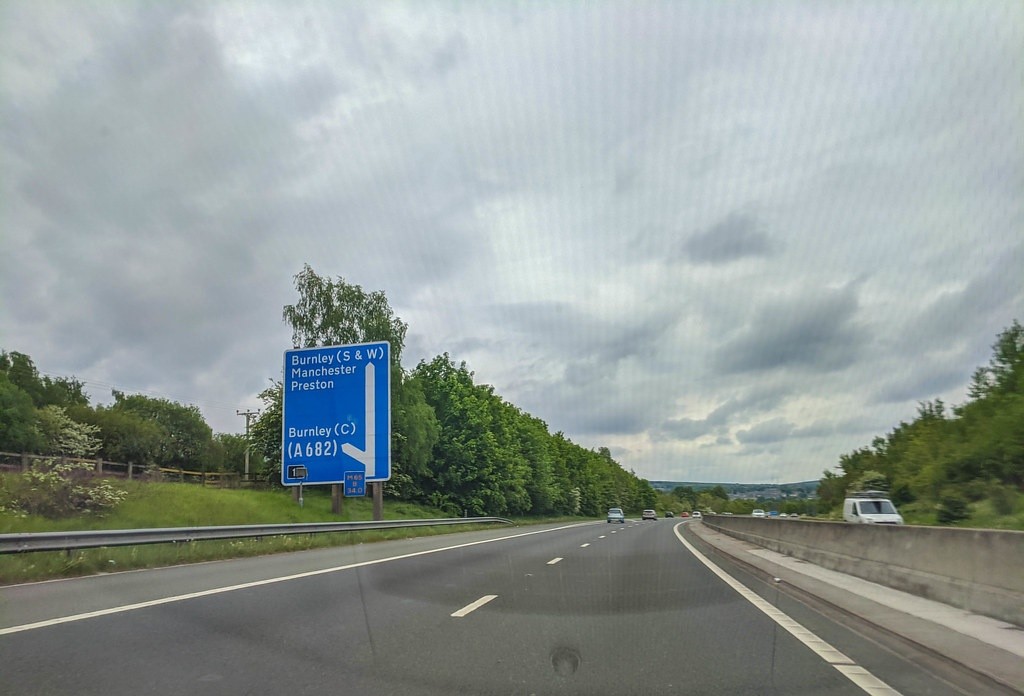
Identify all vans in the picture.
[842,498,904,526]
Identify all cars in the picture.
[666,511,674,518]
[681,512,689,518]
[791,513,798,517]
[692,511,701,519]
[642,510,658,521]
[765,511,778,517]
[607,508,624,524]
[779,513,787,518]
[752,509,765,518]
